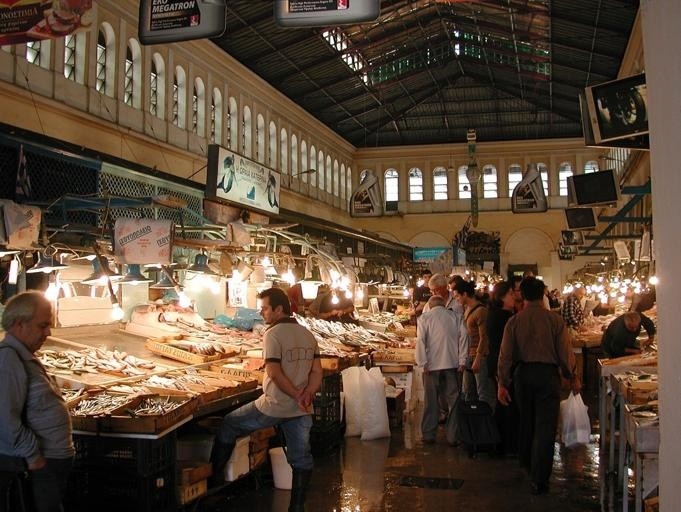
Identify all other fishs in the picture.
[34,346,207,416]
[166,335,228,358]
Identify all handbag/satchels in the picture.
[1,455,35,511]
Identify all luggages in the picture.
[456,366,499,457]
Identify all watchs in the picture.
[466,129,482,228]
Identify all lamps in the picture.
[281,168,318,186]
[0,242,226,322]
[352,246,413,289]
[562,243,660,303]
[596,154,626,163]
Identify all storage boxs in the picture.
[316,321,425,449]
[599,348,660,453]
[66,335,279,505]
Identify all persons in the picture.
[411,269,585,497]
[593,285,656,436]
[0,293,78,511]
[309,284,361,326]
[206,289,324,512]
